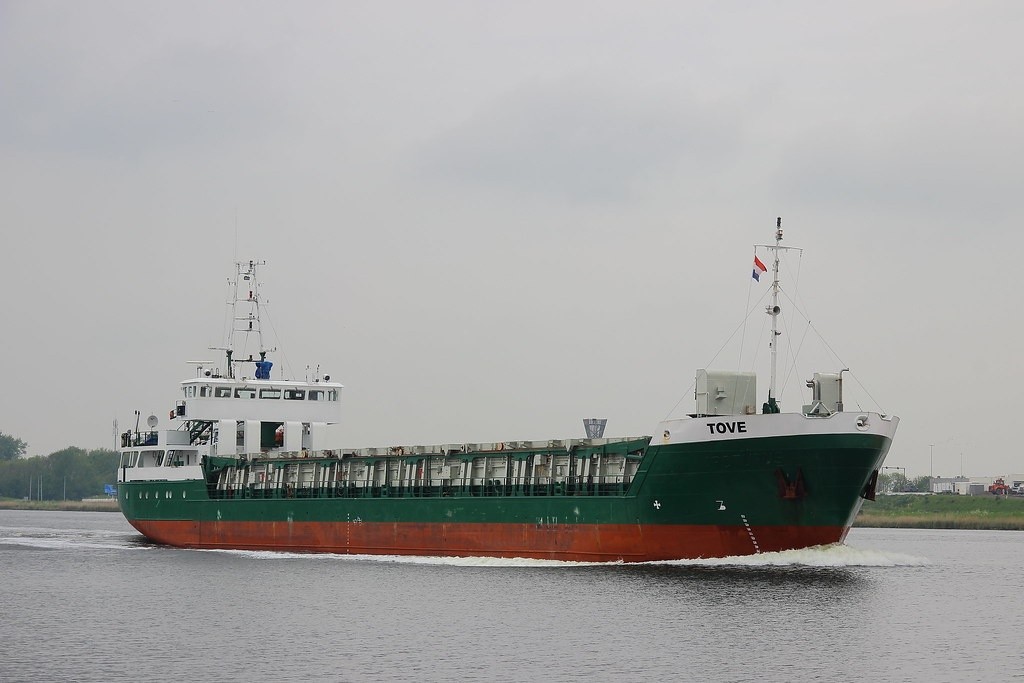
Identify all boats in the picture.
[115,214,899,566]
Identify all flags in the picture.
[752,255,767,283]
